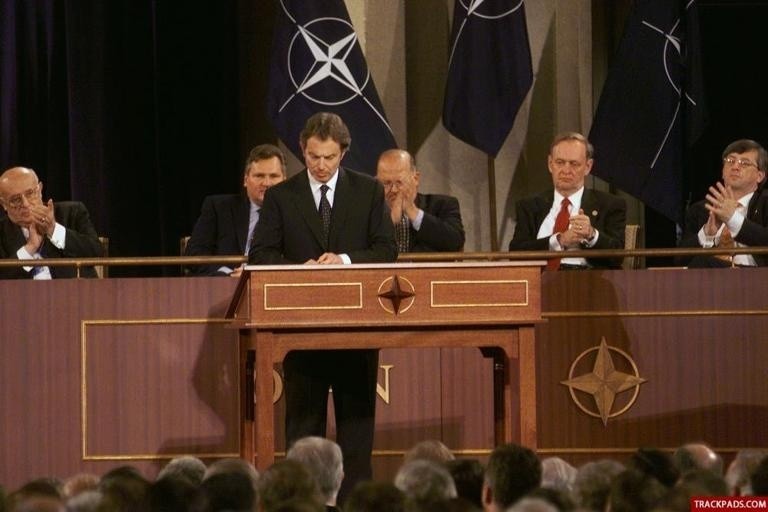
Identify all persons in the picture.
[182,142,290,277]
[244,112,400,500]
[371,147,466,264]
[1,166,107,282]
[685,138,768,270]
[0,429,767,511]
[507,129,629,273]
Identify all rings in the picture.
[576,225,583,230]
[39,216,47,223]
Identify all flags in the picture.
[265,1,404,178]
[584,1,741,225]
[435,0,535,156]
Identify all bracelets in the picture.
[578,236,596,246]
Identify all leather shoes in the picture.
[713,223,732,261]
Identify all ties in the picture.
[544,198,571,271]
[396,215,410,252]
[318,185,332,243]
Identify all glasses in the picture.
[723,157,758,168]
